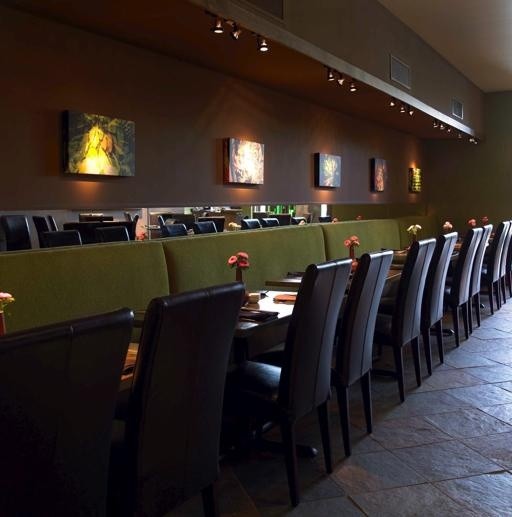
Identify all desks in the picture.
[193,209,243,231]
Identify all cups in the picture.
[247,292,260,303]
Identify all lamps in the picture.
[213,18,481,146]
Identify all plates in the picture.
[229,222,241,227]
[146,224,161,229]
[272,293,297,302]
